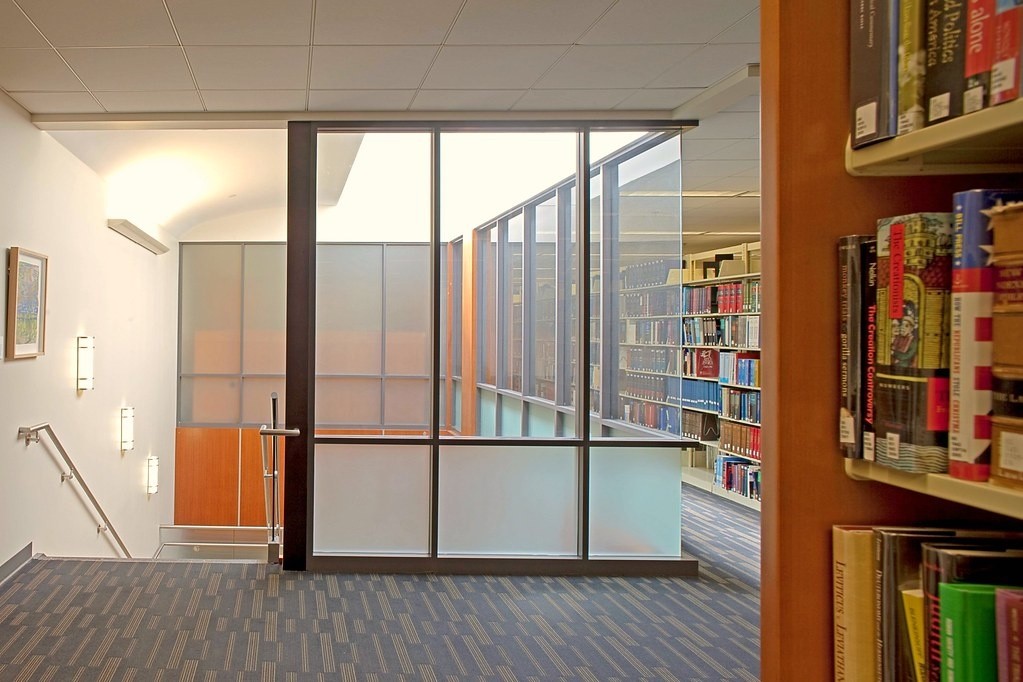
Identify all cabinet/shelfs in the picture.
[512,272,761,512]
[760,0,1023,682]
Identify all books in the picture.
[848,2,1023,150]
[832,524,1023,682]
[837,189,1022,484]
[510,253,759,502]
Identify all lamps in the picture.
[121,407,134,452]
[77,337,95,390]
[148,456,159,494]
[104,217,172,258]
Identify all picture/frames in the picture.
[6,247,48,359]
